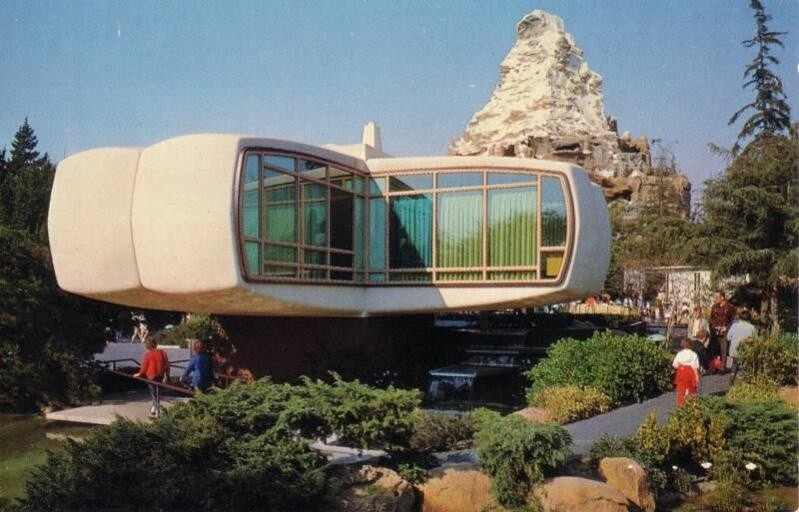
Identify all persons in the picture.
[692,330,725,375]
[126,309,144,342]
[727,309,758,387]
[132,336,171,415]
[137,313,151,341]
[687,306,710,350]
[711,289,735,371]
[672,337,701,406]
[178,339,218,394]
